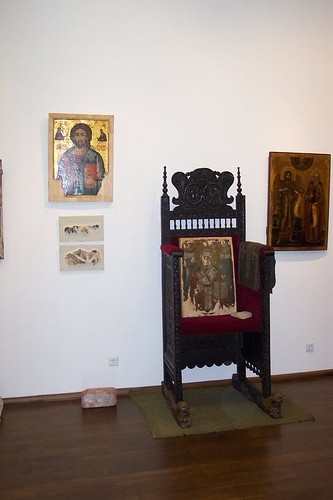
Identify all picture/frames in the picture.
[267,151,331,251]
[48,113,114,203]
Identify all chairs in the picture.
[160,165,284,429]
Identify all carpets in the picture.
[128,383,315,438]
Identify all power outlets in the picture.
[108,356,118,366]
